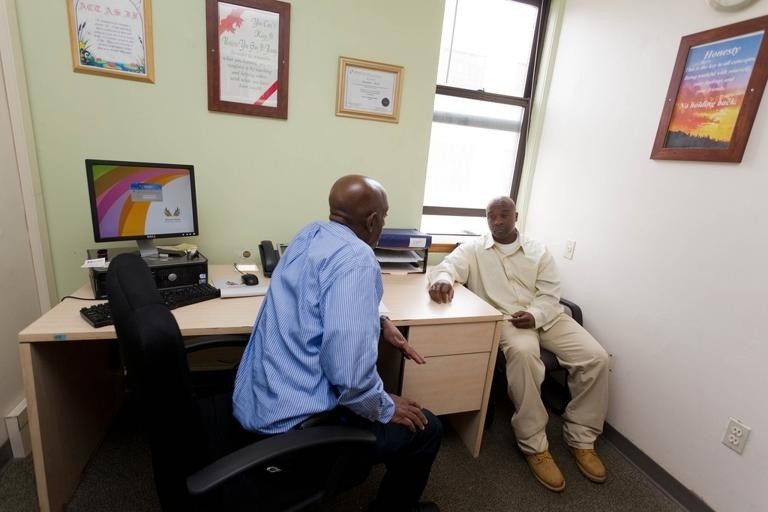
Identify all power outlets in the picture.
[721,417,752,455]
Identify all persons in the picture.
[428,196,611,493]
[233,174,441,512]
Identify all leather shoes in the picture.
[559,437,608,484]
[526,450,566,492]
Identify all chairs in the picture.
[486,296,584,429]
[101,252,404,511]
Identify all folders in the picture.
[376,229,432,247]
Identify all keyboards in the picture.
[79,281,220,329]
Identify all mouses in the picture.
[241,273,259,286]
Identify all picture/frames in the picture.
[649,11,766,164]
[202,1,293,121]
[333,55,405,126]
[64,1,158,84]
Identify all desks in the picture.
[15,262,504,511]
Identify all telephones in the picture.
[258,241,290,278]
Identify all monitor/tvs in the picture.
[84,158,200,257]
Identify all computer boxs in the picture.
[85,244,207,297]
